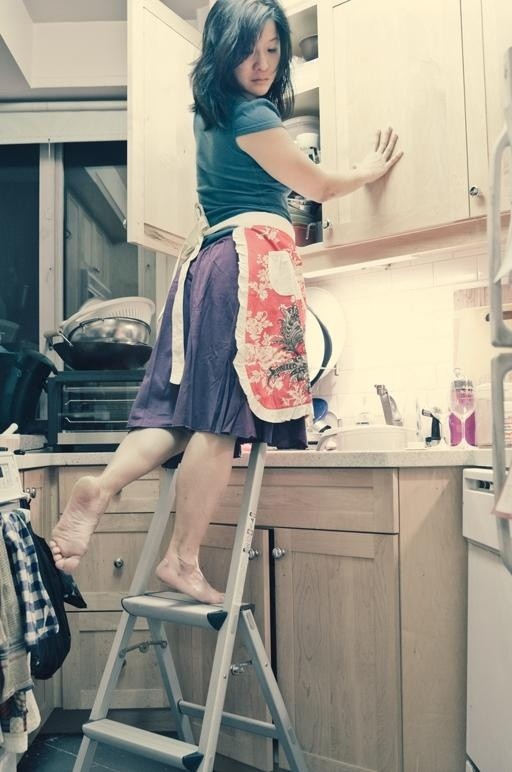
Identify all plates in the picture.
[311,398,328,423]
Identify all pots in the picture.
[42,316,152,371]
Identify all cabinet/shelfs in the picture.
[166,465,463,772]
[53,463,173,714]
[19,467,50,745]
[125,0,468,262]
[458,0,511,220]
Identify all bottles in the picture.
[448,370,477,446]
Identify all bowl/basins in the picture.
[300,36,317,60]
[286,197,318,248]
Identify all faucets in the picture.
[375,382,404,425]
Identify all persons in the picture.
[49,0,402,604]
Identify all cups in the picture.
[295,133,319,164]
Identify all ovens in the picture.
[46,372,145,451]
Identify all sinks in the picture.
[325,423,424,451]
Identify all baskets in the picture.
[62,295,156,331]
